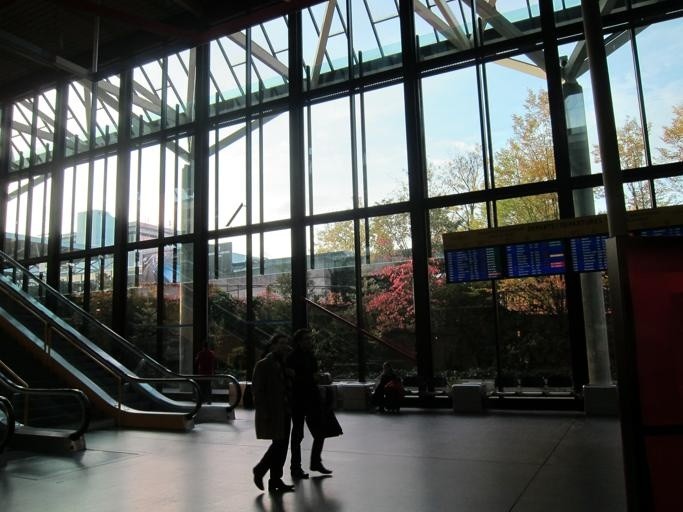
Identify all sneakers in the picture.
[291,469,309,478]
[254,466,264,491]
[310,463,332,474]
[269,479,295,491]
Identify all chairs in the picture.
[490,374,574,396]
[398,376,451,397]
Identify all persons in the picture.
[373,361,396,413]
[251,331,298,492]
[194,340,216,405]
[288,328,343,483]
